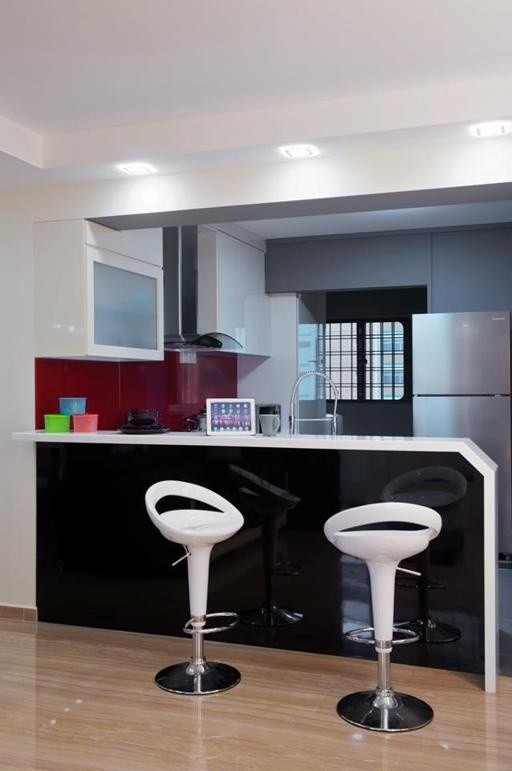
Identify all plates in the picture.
[120,426,170,434]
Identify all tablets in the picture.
[205,397,257,436]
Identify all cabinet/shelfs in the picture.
[31,219,272,360]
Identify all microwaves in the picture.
[256,404,282,433]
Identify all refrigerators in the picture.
[410,312,511,560]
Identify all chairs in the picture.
[323,500,443,736]
[145,478,244,694]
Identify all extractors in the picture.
[162,224,244,354]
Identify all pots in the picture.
[184,409,208,433]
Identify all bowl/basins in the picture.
[128,409,157,425]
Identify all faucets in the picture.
[289,372,338,435]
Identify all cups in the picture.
[43,415,70,433]
[258,415,280,437]
[73,415,99,433]
[58,397,88,415]
